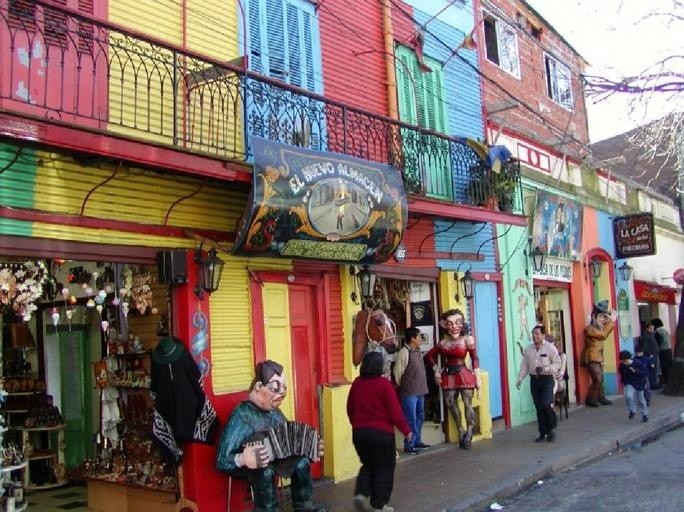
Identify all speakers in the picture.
[156,248,187,285]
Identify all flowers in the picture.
[131,272,155,315]
[1,260,49,325]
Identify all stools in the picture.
[222,471,285,510]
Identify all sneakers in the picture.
[405,448,418,454]
[414,443,431,450]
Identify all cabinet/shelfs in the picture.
[62,349,180,493]
[0,458,30,512]
[0,391,68,489]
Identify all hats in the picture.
[152,337,184,364]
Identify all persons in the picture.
[618,317,673,421]
[426,308,481,449]
[516,325,562,442]
[581,299,617,407]
[346,350,413,511]
[392,328,430,455]
[545,336,566,407]
[220,361,328,512]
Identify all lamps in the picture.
[453,260,476,303]
[582,256,602,286]
[10,320,36,371]
[524,235,546,278]
[193,245,224,299]
[348,265,377,305]
[614,256,632,285]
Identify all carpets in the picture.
[18,477,88,511]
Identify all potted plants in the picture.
[467,164,519,216]
